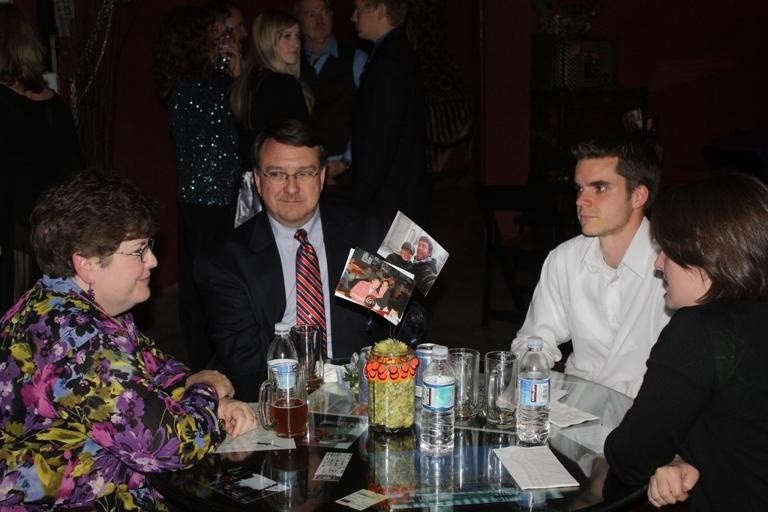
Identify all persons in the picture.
[1,168,259,511]
[1,21,81,313]
[325,0,427,236]
[604,164,768,512]
[226,7,318,169]
[385,241,414,274]
[289,1,369,190]
[166,3,253,346]
[350,276,383,306]
[510,127,677,399]
[193,119,394,403]
[413,237,438,293]
[374,279,390,310]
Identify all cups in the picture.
[484,350,519,425]
[291,324,323,392]
[367,345,416,432]
[259,361,309,439]
[447,347,481,418]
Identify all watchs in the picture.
[338,160,352,172]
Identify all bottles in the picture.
[518,337,550,444]
[414,342,434,411]
[275,468,298,510]
[420,344,455,456]
[359,346,372,406]
[267,324,297,402]
[421,451,454,512]
[517,488,549,512]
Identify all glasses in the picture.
[256,165,322,185]
[113,238,155,263]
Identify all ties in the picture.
[294,229,327,359]
